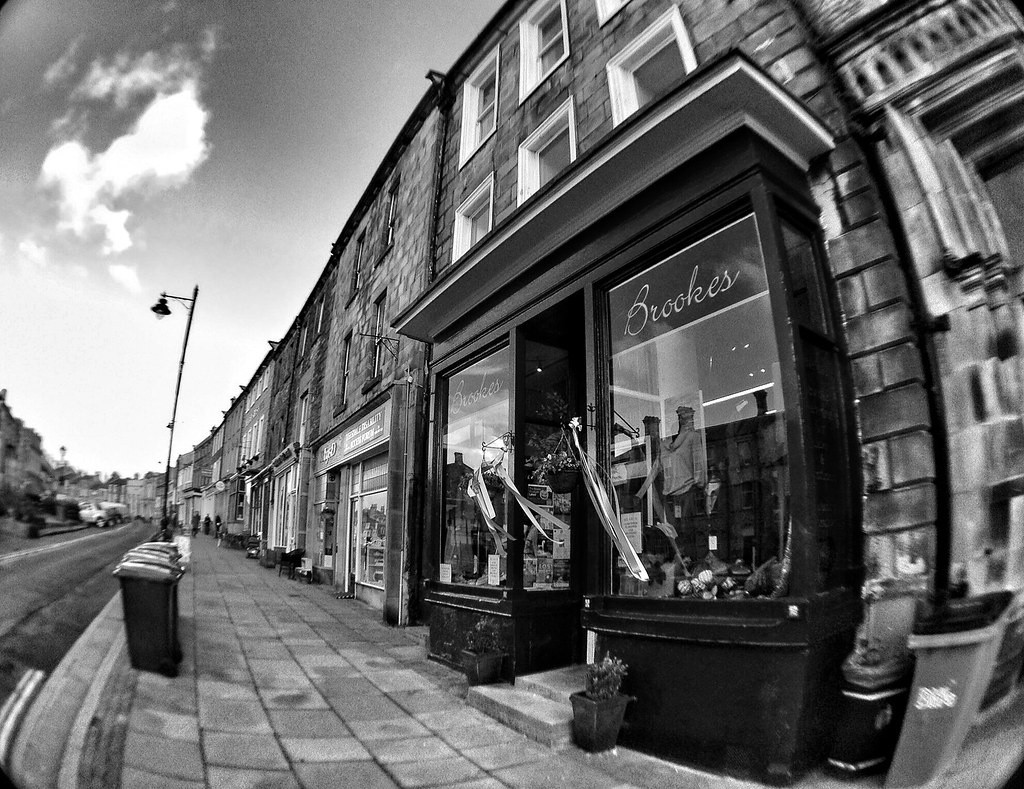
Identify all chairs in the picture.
[278,547,306,579]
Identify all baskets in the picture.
[473,487,504,532]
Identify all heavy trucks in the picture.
[76,498,131,527]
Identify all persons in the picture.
[191,511,201,538]
[204,513,211,535]
[213,515,221,539]
[719,398,780,566]
[635,428,709,559]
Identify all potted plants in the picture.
[569,650,630,753]
[460,614,510,691]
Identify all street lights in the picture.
[144,282,204,543]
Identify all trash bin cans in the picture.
[113,539,187,678]
